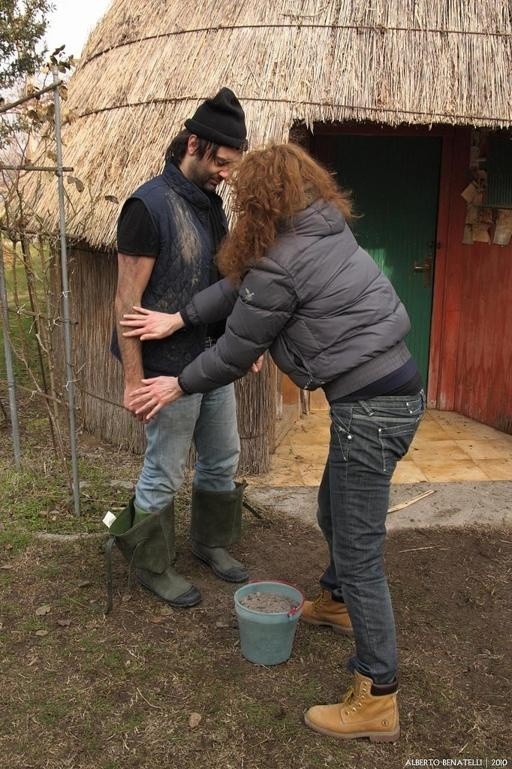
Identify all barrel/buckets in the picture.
[235,578,305,666]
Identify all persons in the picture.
[119,143,425,745]
[108,88,250,608]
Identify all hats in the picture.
[184,87,248,151]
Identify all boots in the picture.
[109,493,203,608]
[300,589,353,635]
[190,480,250,583]
[305,670,400,743]
